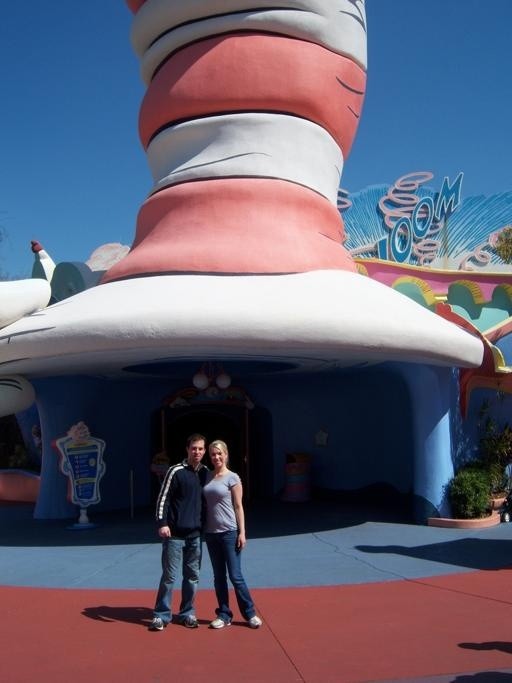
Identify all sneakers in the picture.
[184,615,198,628]
[248,617,263,629]
[149,618,168,631]
[210,618,231,629]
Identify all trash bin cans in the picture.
[284,452,313,502]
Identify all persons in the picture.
[31,424,42,454]
[503,451,512,521]
[148,432,210,631]
[202,438,263,630]
